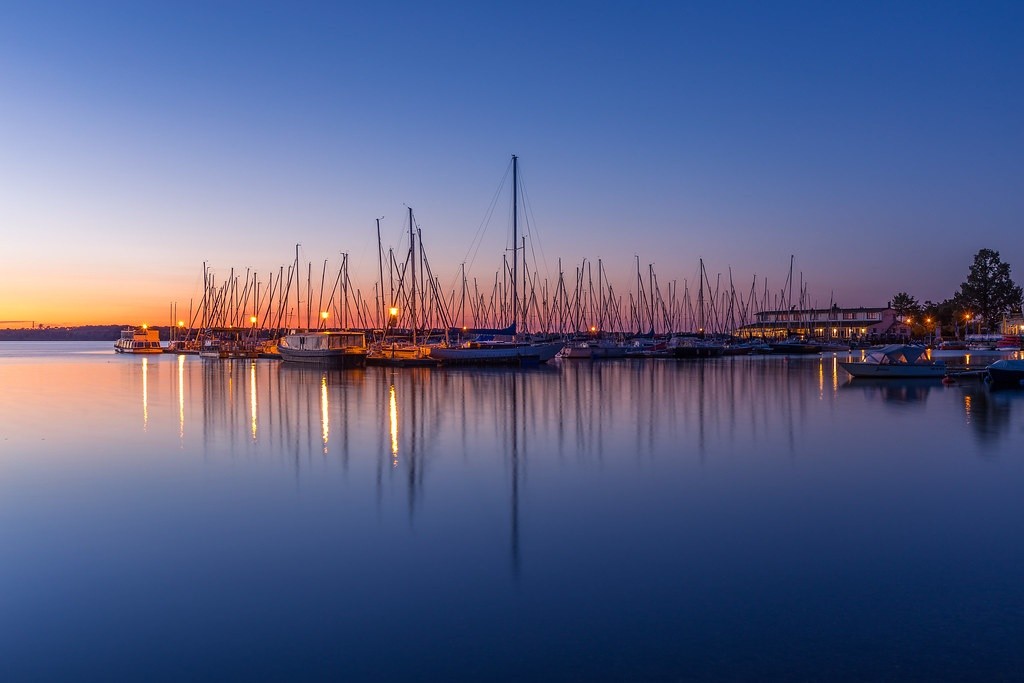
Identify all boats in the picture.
[984,359,1024,389]
[112,327,165,354]
[839,343,949,379]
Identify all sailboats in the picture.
[166,154,851,370]
